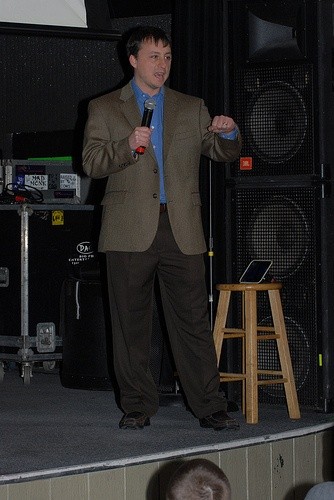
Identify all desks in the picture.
[0,203,99,385]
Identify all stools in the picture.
[212,283,301,427]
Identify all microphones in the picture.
[135,98,156,155]
[0,194,30,203]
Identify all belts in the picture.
[159,201,167,211]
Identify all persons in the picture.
[166,458,232,500]
[82,24,243,431]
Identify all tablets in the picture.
[239,260,273,284]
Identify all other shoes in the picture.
[120,413,150,429]
[200,414,240,432]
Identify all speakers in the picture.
[61,279,179,397]
[215,181,334,414]
[214,0,334,183]
